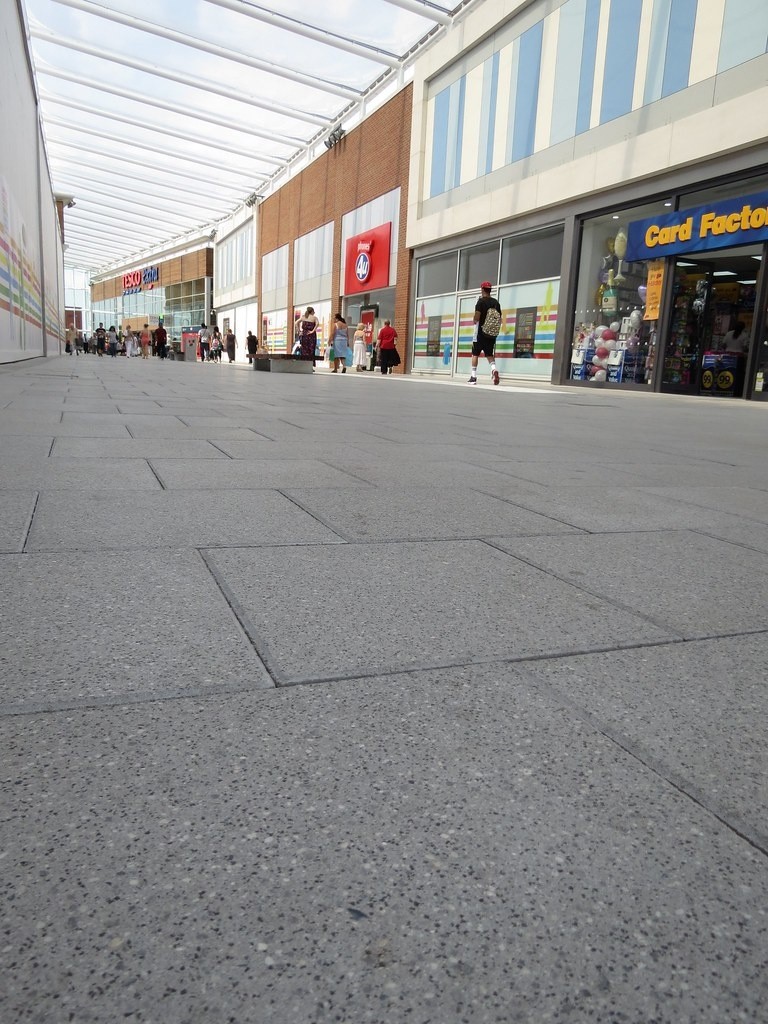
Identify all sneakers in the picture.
[467,377,476,384]
[492,369,499,386]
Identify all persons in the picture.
[354,323,367,372]
[467,281,502,384]
[295,307,319,371]
[68,323,168,360]
[723,321,749,354]
[328,313,350,374]
[198,324,238,364]
[245,331,258,364]
[375,320,401,375]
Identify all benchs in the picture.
[168,352,184,361]
[246,354,324,374]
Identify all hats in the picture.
[481,281,492,289]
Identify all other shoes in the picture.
[382,372,387,375]
[332,369,337,373]
[357,368,363,373]
[389,367,392,375]
[342,367,346,374]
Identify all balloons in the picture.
[590,286,648,382]
[696,279,707,297]
[693,298,705,316]
[712,287,717,294]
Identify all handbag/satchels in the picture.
[123,330,128,336]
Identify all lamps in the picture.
[324,123,346,149]
[244,192,265,207]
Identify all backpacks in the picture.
[480,299,500,336]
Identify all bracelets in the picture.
[376,346,378,348]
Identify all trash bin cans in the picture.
[172,341,184,361]
